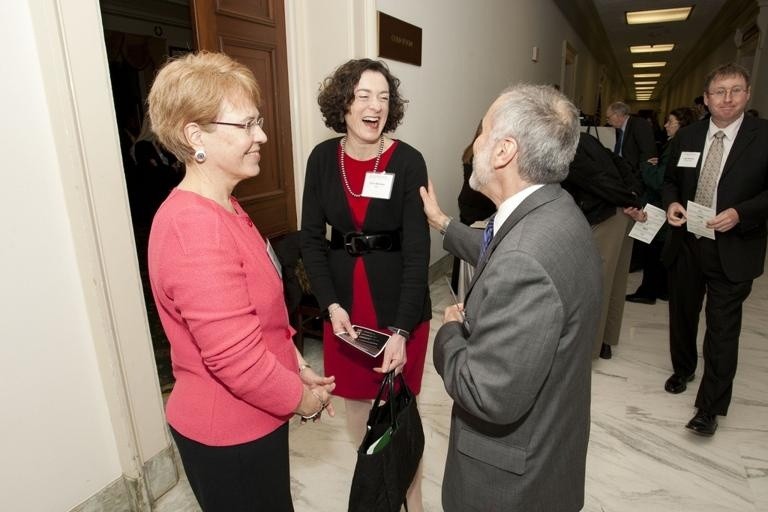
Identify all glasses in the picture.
[606,112,617,122]
[708,85,749,98]
[207,116,266,130]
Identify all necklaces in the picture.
[340,135,384,199]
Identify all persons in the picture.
[658,61,768,437]
[450,120,497,295]
[142,47,338,512]
[295,58,433,511]
[127,109,185,203]
[747,108,760,117]
[416,80,606,510]
[560,96,710,360]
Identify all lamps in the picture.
[625,6,692,25]
[631,61,667,101]
[630,44,675,54]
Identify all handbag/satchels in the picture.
[338,365,432,512]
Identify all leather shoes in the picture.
[599,341,612,360]
[684,406,720,438]
[663,369,696,394]
[625,285,669,306]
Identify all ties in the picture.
[692,131,727,241]
[475,215,497,272]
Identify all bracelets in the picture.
[392,324,411,338]
[300,392,326,421]
[299,365,311,372]
[439,215,454,238]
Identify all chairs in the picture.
[267,229,323,358]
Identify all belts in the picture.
[327,226,404,258]
